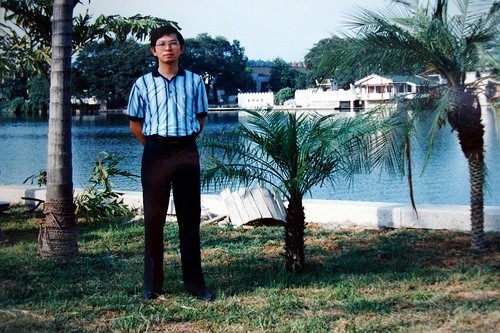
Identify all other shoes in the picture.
[142,285,164,299]
[196,287,214,301]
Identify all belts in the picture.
[156,136,193,144]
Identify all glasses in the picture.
[155,40,181,48]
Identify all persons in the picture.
[127,26,217,300]
